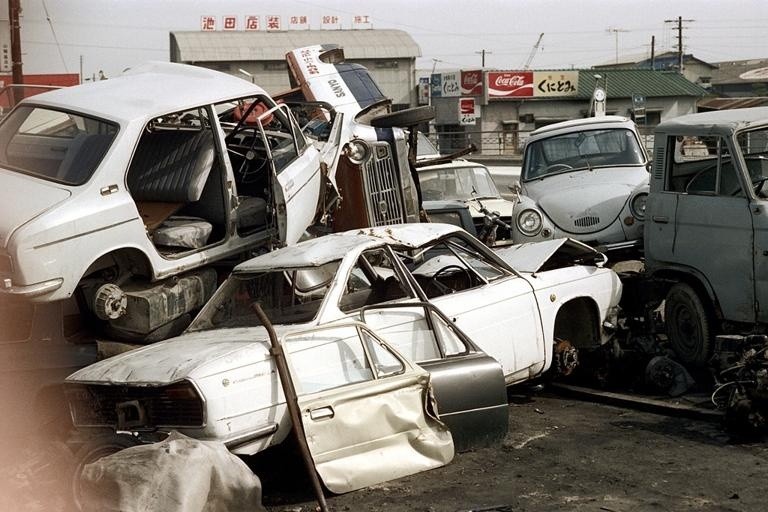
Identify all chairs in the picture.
[182,153,267,227]
